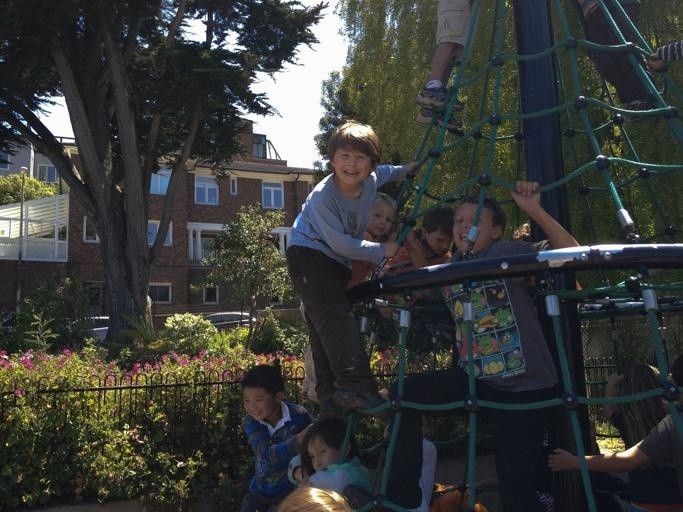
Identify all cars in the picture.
[63,316,110,342]
[204,312,257,329]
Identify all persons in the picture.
[415,1,473,138]
[241,364,372,512]
[546,357,682,511]
[348,180,581,511]
[285,120,421,419]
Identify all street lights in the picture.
[15,165,28,317]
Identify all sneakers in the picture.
[331,388,393,420]
[413,84,464,113]
[411,107,465,131]
[316,394,352,423]
